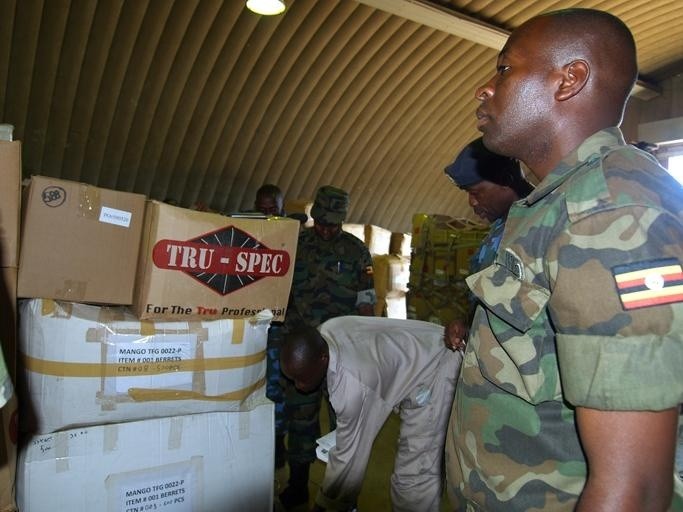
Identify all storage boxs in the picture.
[0,140,304,508]
[367,204,494,351]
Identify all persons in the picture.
[279,314,465,512]
[444,137,537,351]
[444,8,683,512]
[279,186,378,505]
[254,184,285,469]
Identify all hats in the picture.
[445,137,524,189]
[311,185,349,225]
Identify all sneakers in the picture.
[279,488,309,509]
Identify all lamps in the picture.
[242,0,285,19]
